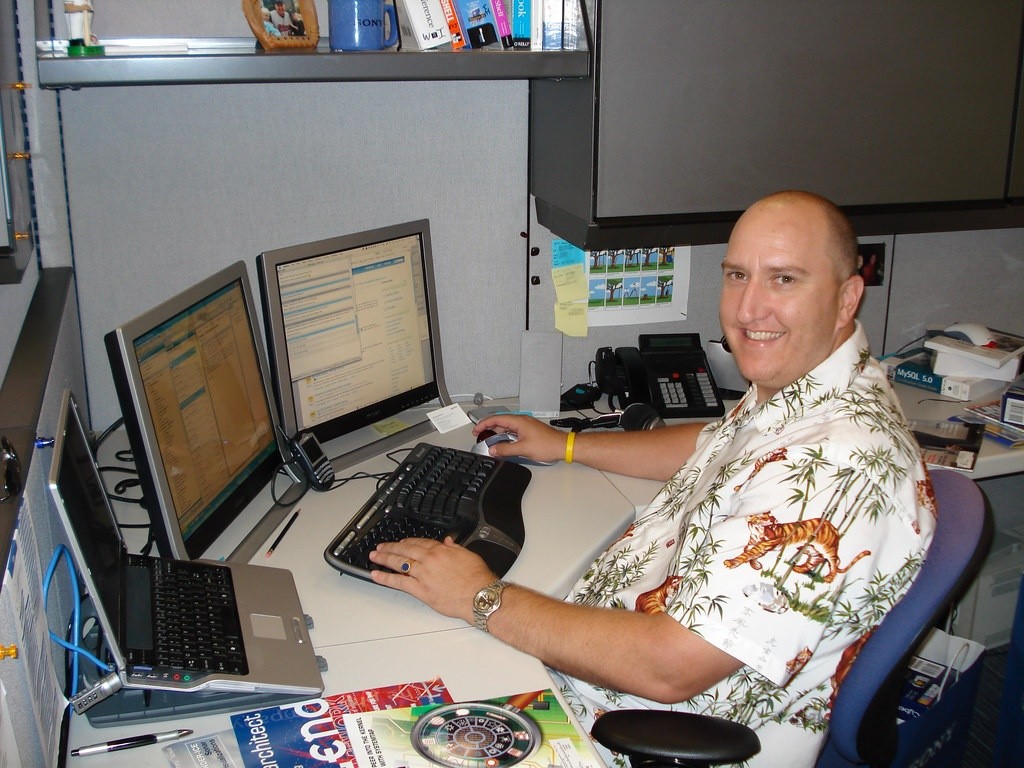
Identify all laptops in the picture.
[47,388,324,695]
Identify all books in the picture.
[874,321,1024,473]
[394,0,591,52]
[342,689,606,768]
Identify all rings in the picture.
[402,558,416,576]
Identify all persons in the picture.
[258,0,305,39]
[856,255,882,283]
[369,190,938,768]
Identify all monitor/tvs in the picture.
[104,219,459,564]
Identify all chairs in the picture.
[818,466,995,768]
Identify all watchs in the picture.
[473,579,512,633]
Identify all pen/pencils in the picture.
[265,508,303,559]
[70,728,194,757]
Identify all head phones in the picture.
[571,402,666,433]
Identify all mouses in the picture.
[470,428,558,465]
[944,321,996,347]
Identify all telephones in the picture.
[618,330,726,419]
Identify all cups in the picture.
[328,0,397,51]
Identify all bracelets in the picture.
[565,432,575,464]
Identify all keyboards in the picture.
[324,442,532,590]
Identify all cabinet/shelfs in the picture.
[38,0,1024,433]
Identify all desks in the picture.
[64,377,1024,768]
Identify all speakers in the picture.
[519,331,563,419]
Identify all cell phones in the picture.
[276,424,335,491]
